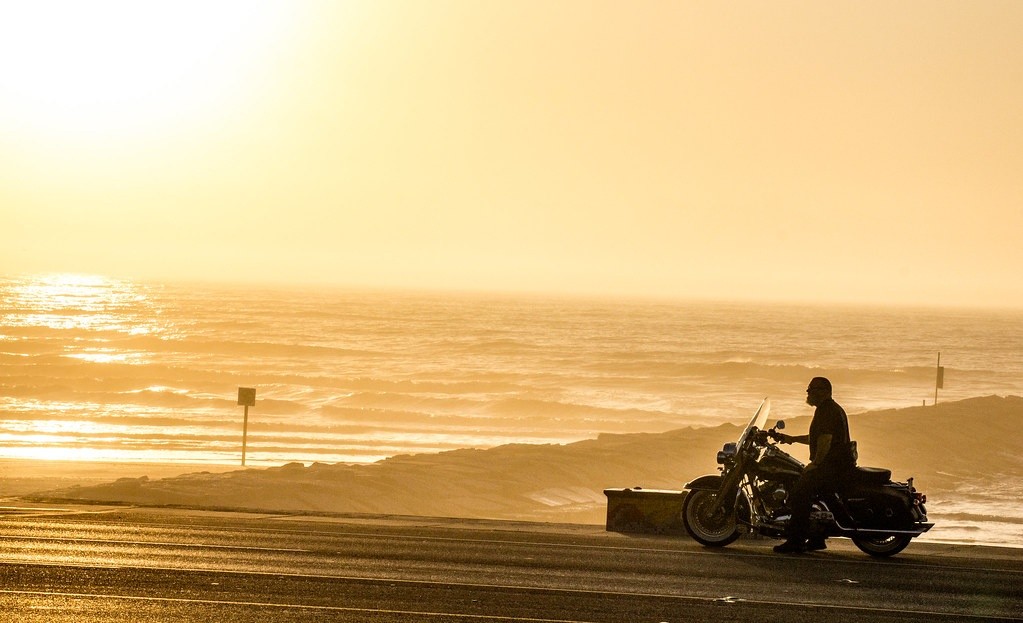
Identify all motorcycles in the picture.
[680,395,935,559]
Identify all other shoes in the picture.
[804,541,828,550]
[774,540,806,553]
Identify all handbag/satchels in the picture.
[834,441,858,463]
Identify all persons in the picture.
[773,376,856,554]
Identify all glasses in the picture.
[808,386,825,391]
